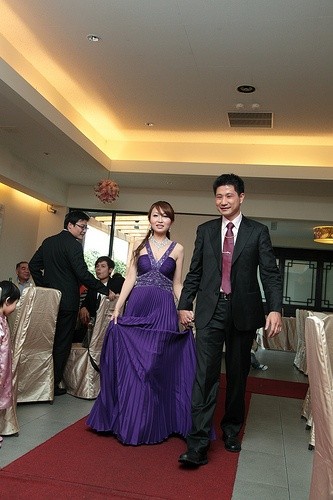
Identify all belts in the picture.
[217,293,231,300]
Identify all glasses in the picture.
[75,223,88,230]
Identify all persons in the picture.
[87,200,215,445]
[178,172,283,465]
[73,256,122,343]
[29,210,115,396]
[14,261,34,297]
[0,280,20,449]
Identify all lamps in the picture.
[313,226,333,244]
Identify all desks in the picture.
[266,315,298,352]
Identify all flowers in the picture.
[95,178,121,205]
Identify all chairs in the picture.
[296,309,333,500]
[65,296,118,399]
[7,287,62,405]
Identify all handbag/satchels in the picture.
[83,317,95,348]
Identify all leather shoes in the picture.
[178,453,208,465]
[222,433,241,452]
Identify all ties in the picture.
[222,222,235,295]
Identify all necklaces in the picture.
[149,236,169,250]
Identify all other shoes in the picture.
[258,363,269,370]
[54,388,66,396]
